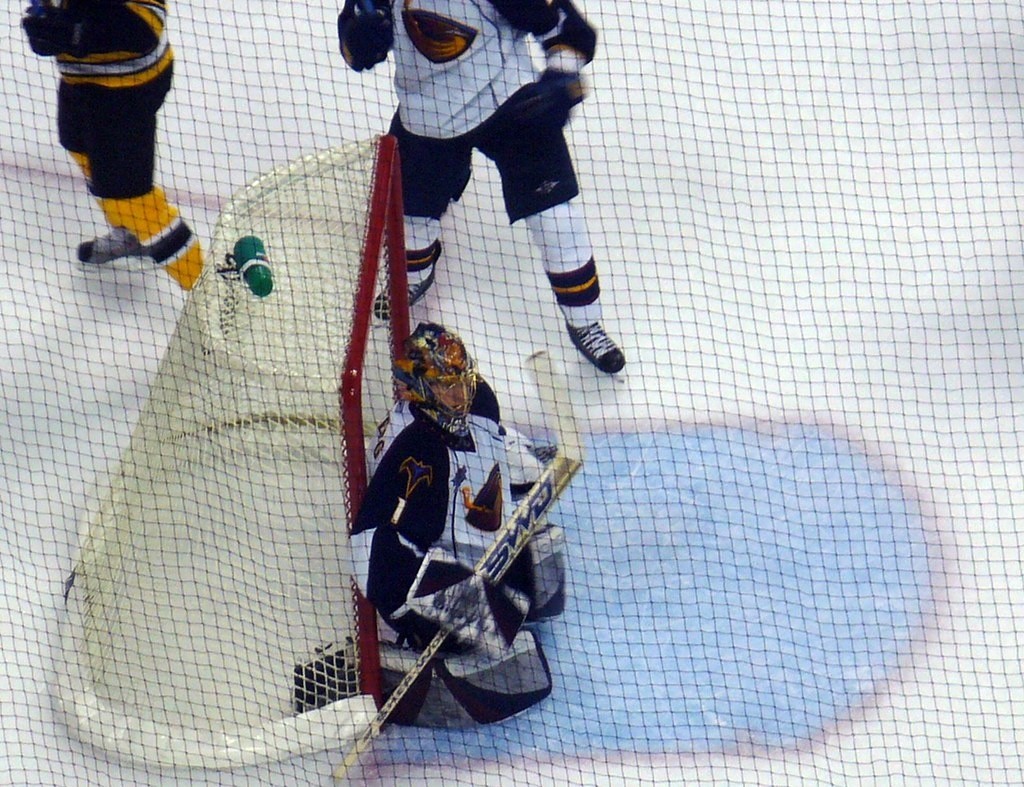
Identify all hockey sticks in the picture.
[321,350,585,786]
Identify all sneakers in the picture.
[567,325,625,381]
[76,224,159,272]
[374,239,441,321]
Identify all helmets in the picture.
[403,322,477,438]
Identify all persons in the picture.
[293,322,567,732]
[336,1,626,373]
[21,0,205,291]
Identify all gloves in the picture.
[341,9,391,71]
[505,66,581,133]
[22,5,90,58]
